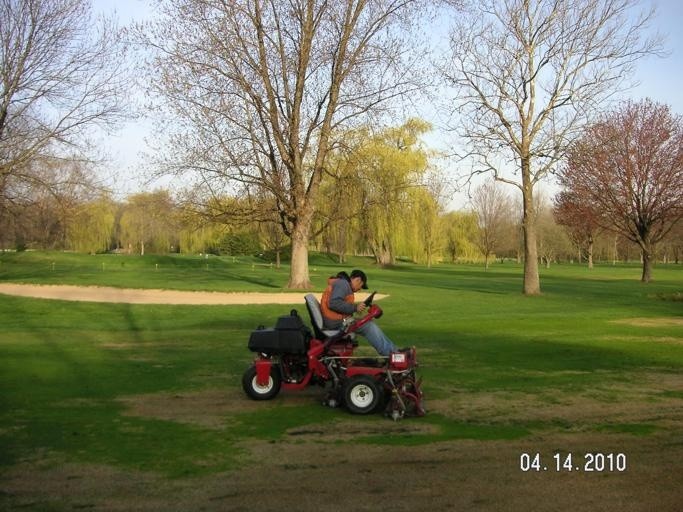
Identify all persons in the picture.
[320,269,412,365]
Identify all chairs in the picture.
[302,292,355,344]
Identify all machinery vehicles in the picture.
[243,291,426,421]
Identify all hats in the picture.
[349,270,369,289]
